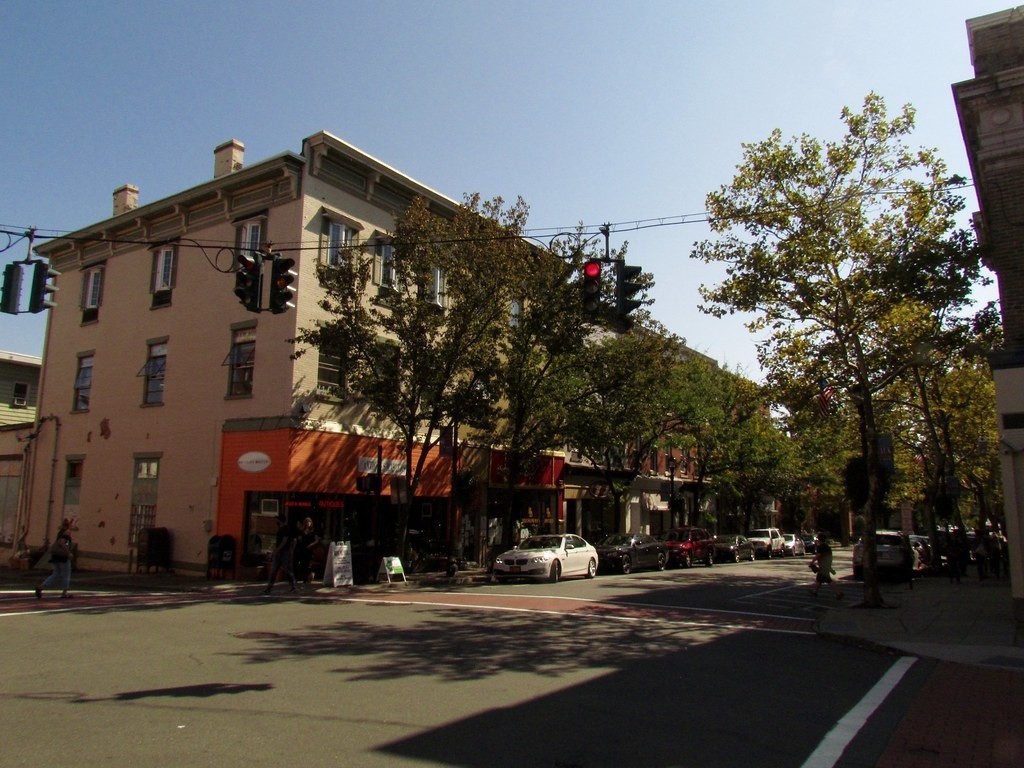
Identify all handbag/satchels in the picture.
[51,543,66,562]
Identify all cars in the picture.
[594,534,669,574]
[493,534,599,583]
[713,534,756,563]
[783,534,819,557]
[852,529,932,582]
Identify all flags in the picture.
[818,375,837,420]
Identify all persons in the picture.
[33,518,74,599]
[943,529,1009,587]
[259,515,319,595]
[808,534,843,601]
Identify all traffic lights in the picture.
[232,253,264,313]
[0,263,24,315]
[616,263,642,331]
[29,263,62,314]
[582,261,601,317]
[269,258,294,315]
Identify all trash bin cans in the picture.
[137,526,172,571]
[205,533,238,580]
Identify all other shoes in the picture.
[35,585,42,598]
[259,588,271,595]
[61,593,72,598]
[289,587,296,593]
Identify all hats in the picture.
[62,518,79,531]
[274,514,287,522]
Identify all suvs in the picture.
[658,526,715,568]
[746,528,785,559]
